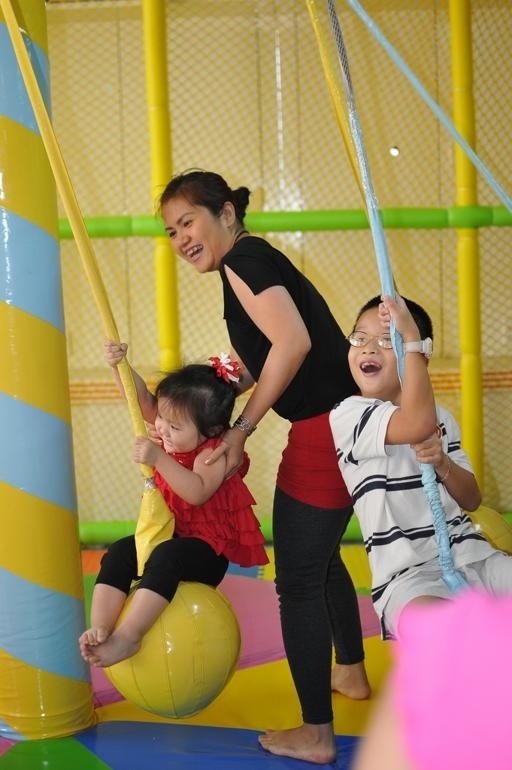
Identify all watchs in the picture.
[404,337,435,359]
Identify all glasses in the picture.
[347,331,392,349]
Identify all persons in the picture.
[158,171,372,766]
[328,295,511,641]
[79,340,272,669]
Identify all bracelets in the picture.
[233,416,257,437]
